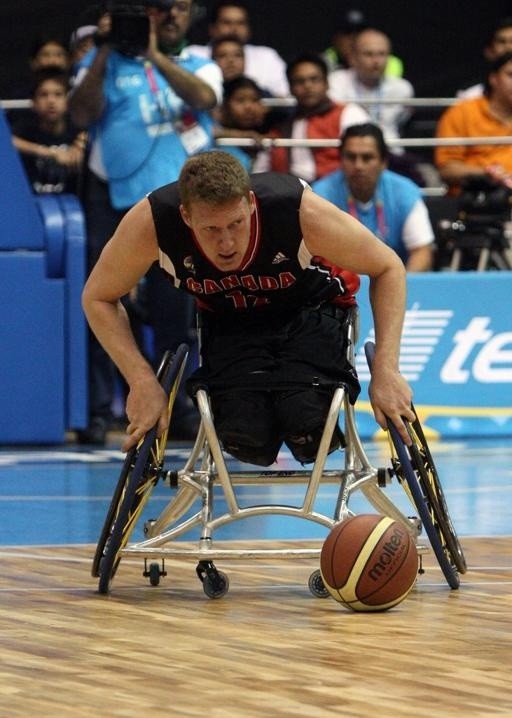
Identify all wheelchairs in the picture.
[90,313,470,601]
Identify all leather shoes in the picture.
[77,417,106,446]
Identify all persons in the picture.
[3,0,511,278]
[55,0,228,446]
[79,150,417,467]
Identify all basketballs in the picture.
[319,513,418,613]
